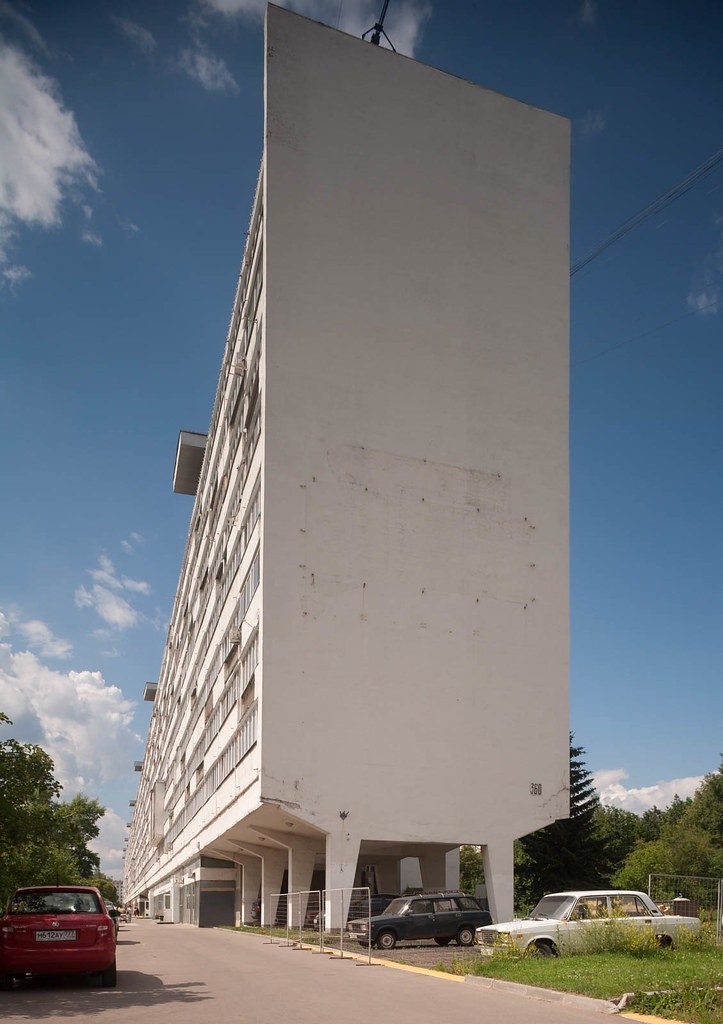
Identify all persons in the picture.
[127,905,132,922]
[74,898,90,912]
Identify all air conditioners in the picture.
[229,626,238,640]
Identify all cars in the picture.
[345,892,492,950]
[0,886,120,990]
[472,890,703,961]
[73,899,129,942]
[251,890,433,933]
